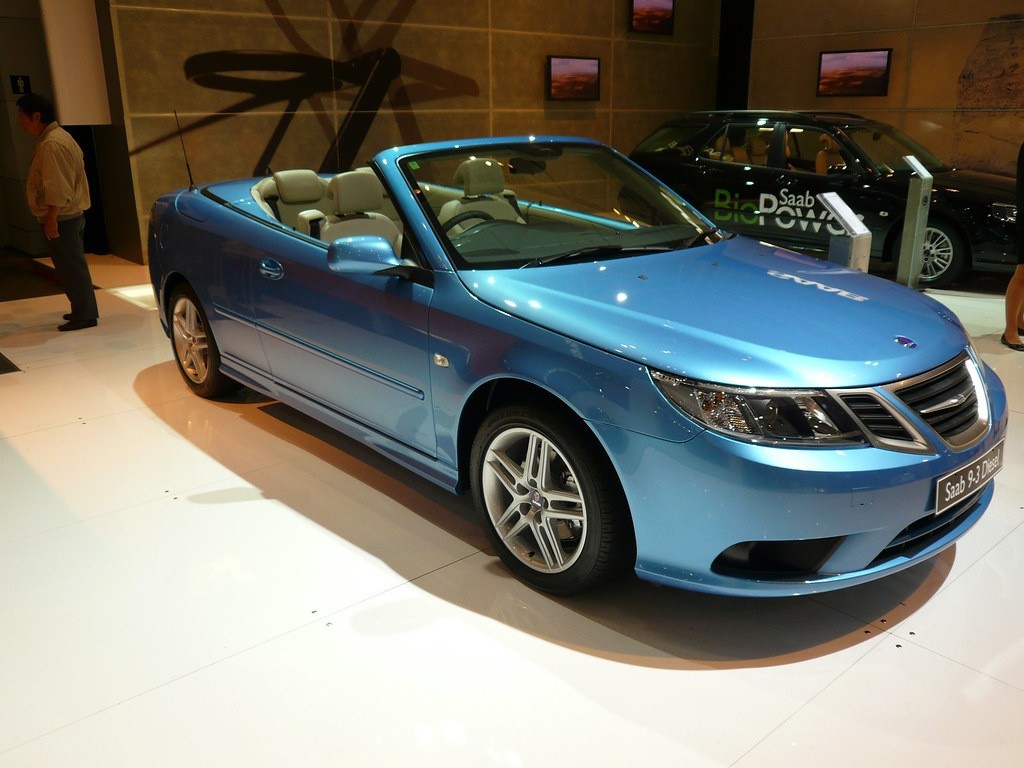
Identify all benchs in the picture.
[265,166,403,241]
[711,128,790,166]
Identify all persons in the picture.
[1001,143,1024,352]
[15,92,100,331]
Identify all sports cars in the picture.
[147,134,1010,603]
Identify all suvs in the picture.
[619,108,1019,289]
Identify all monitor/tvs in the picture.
[815,48,894,97]
[545,55,601,101]
[628,0,675,37]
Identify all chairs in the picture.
[438,158,527,238]
[317,171,404,261]
[815,134,845,175]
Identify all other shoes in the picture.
[63,310,98,320]
[1018,328,1024,335]
[57,318,97,331]
[1000,334,1024,351]
